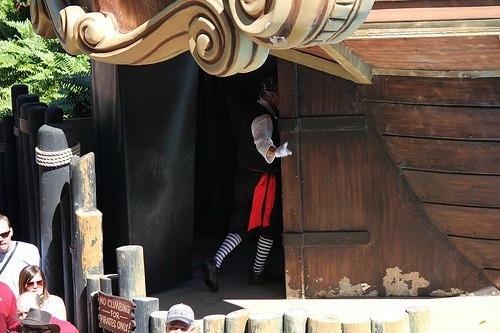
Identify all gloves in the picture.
[275,142,292,158]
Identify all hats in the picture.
[12,308,60,333]
[262,78,278,91]
[166,303,194,324]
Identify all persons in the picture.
[15,292,79,333]
[165,303,194,333]
[12,308,60,333]
[0,214,40,299]
[0,280,22,333]
[16,265,67,320]
[201,77,293,291]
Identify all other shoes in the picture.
[201,258,220,291]
[249,270,281,285]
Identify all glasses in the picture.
[0,231,10,238]
[27,280,44,288]
[23,325,50,333]
[169,322,190,331]
